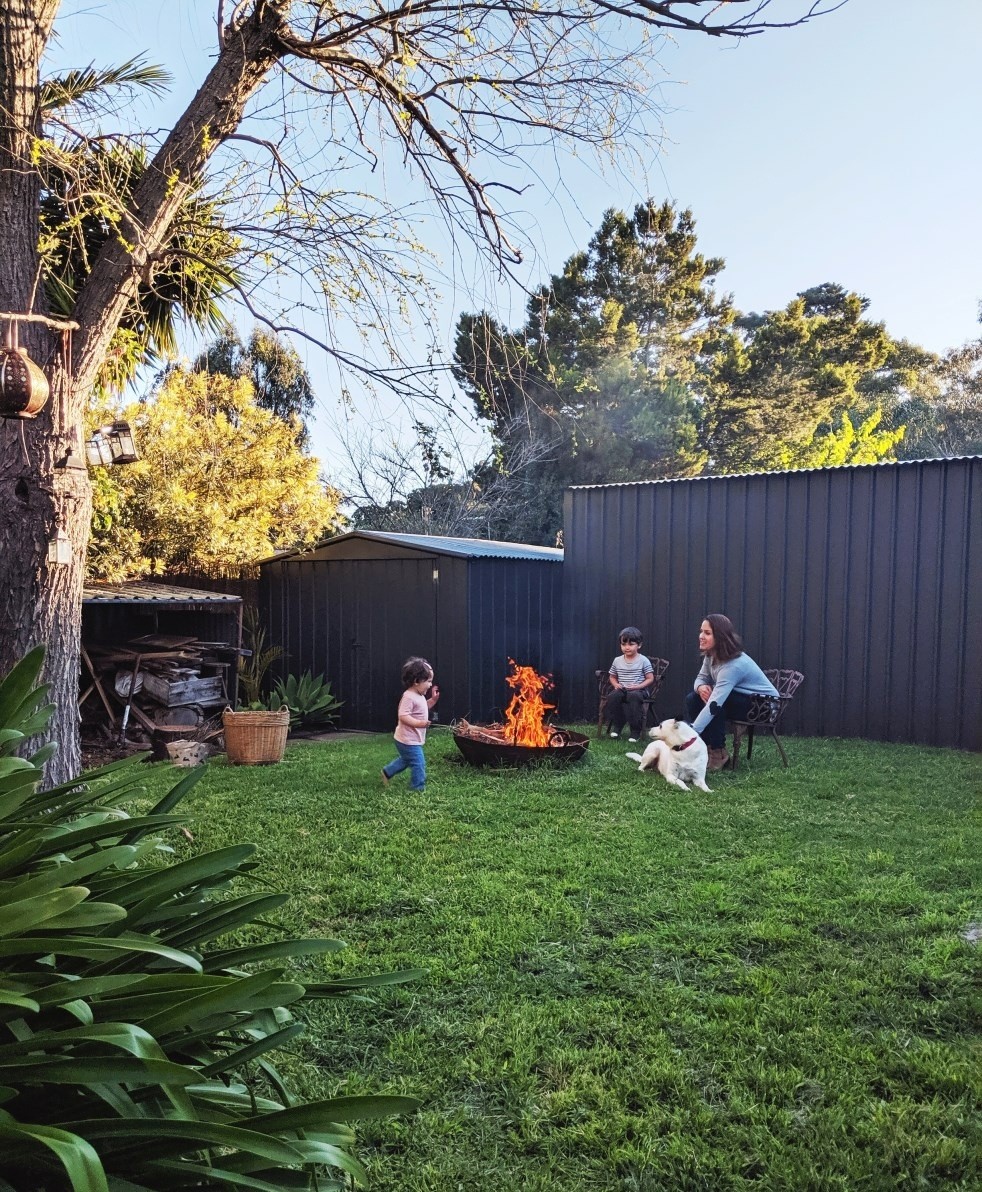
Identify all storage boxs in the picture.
[142,672,222,708]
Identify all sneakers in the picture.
[628,732,640,743]
[610,725,623,738]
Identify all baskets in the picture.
[222,705,291,765]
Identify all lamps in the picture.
[85,411,140,466]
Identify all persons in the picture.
[686,612,781,773]
[605,626,655,743]
[379,656,440,792]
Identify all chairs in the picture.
[724,669,805,769]
[594,656,670,739]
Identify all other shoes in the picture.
[707,743,712,769]
[708,746,731,773]
[381,768,390,785]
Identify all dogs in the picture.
[625,717,714,793]
